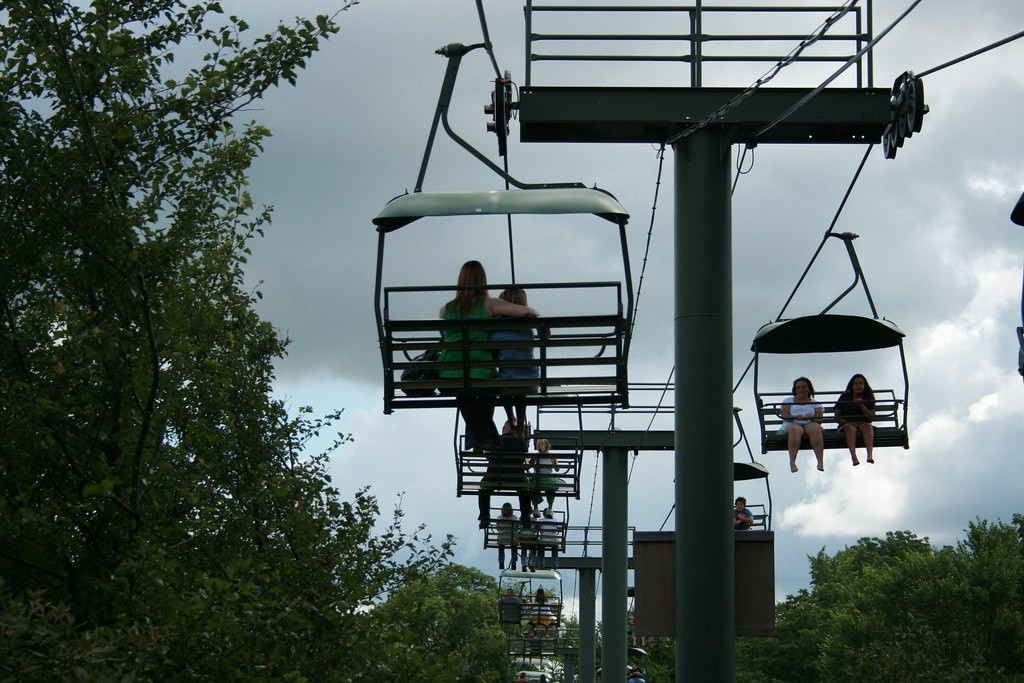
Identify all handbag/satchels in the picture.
[400,350,438,396]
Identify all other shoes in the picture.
[534,507,541,517]
[529,565,535,572]
[512,561,517,570]
[522,565,527,572]
[499,561,504,568]
[479,520,490,529]
[547,508,554,516]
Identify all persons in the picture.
[478,419,531,530]
[517,673,528,683]
[628,661,646,683]
[734,496,754,532]
[776,377,824,472]
[496,584,552,639]
[519,508,558,572]
[530,439,560,520]
[648,637,670,648]
[540,674,546,683]
[496,503,519,570]
[512,630,544,667]
[490,289,539,446]
[629,613,645,645]
[834,374,876,465]
[439,261,551,451]
